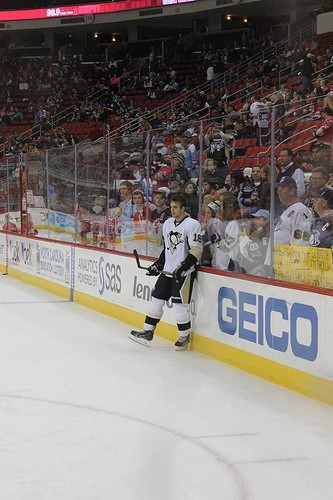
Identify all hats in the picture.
[208,200,222,213]
[155,189,166,196]
[252,209,270,219]
[309,187,333,204]
[244,167,252,177]
[276,176,296,189]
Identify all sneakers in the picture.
[174,335,190,351]
[129,329,154,347]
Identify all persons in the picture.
[139,39,333,147]
[129,196,203,351]
[110,131,269,271]
[310,142,333,172]
[310,188,333,247]
[304,166,329,208]
[0,54,144,159]
[264,176,312,275]
[240,210,270,277]
[275,148,305,199]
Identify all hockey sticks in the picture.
[134,248,174,275]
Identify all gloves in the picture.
[146,263,161,276]
[173,262,189,283]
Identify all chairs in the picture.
[0,32,333,192]
